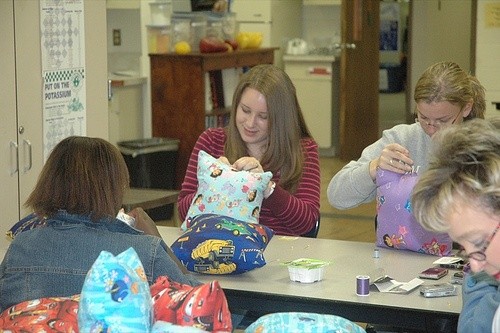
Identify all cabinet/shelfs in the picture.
[230,0,304,69]
[282,54,341,159]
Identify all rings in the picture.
[390,159,393,165]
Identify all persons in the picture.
[410,115,500,333]
[326,62,486,250]
[0,136,202,312]
[177,64,321,238]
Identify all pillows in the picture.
[181,150,273,232]
[150,275,233,333]
[169,213,276,276]
[375,165,455,256]
[77,247,154,333]
[0,293,81,333]
[245,311,367,333]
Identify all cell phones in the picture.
[420,283,457,298]
[419,267,448,279]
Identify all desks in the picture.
[123,188,182,229]
[157,227,468,333]
[149,46,281,189]
